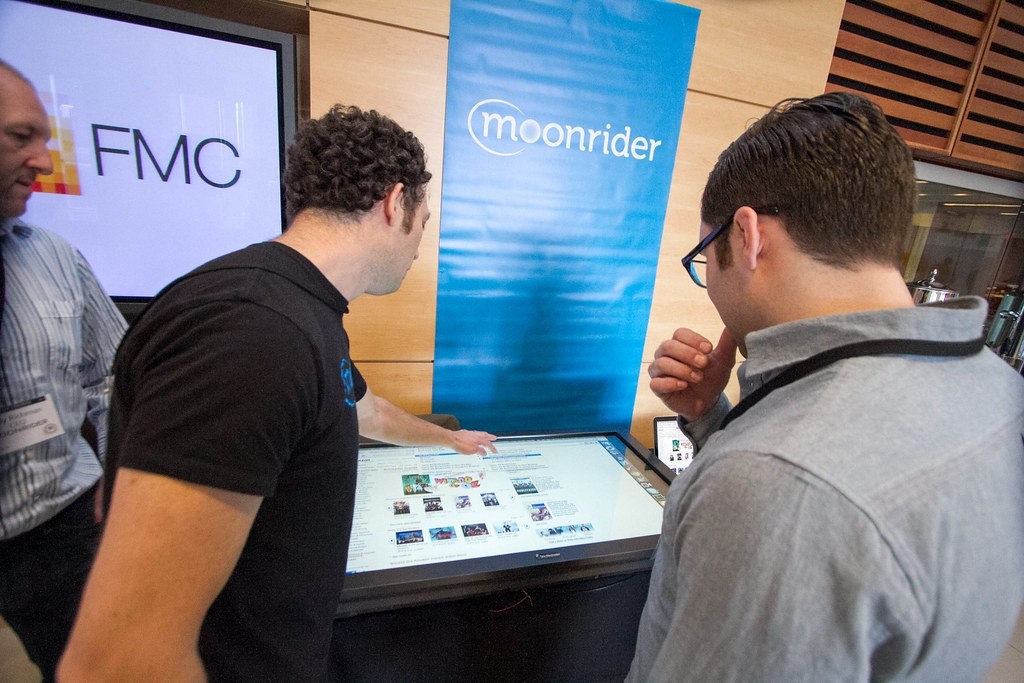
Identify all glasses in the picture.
[681,205,780,289]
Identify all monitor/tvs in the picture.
[0,0,298,316]
[338,431,680,602]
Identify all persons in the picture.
[1,55,132,683]
[54,102,501,682]
[622,93,1024,682]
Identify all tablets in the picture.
[653,415,694,475]
